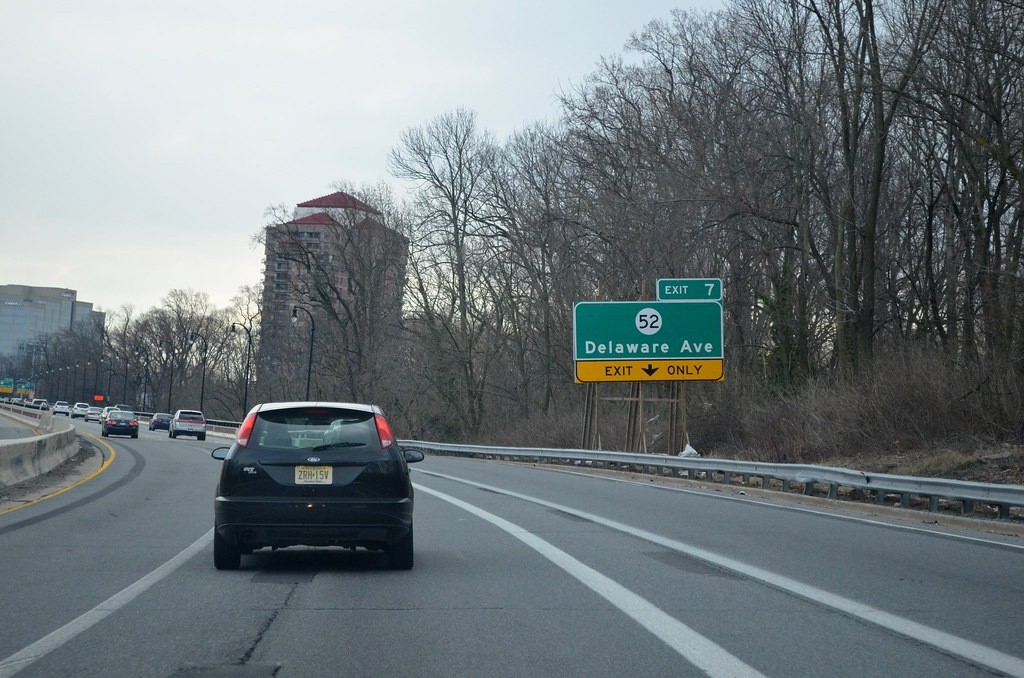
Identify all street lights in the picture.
[76,360,86,402]
[230,323,252,420]
[134,345,148,412]
[190,331,207,412]
[158,340,175,413]
[87,358,98,407]
[26,366,68,403]
[291,304,315,402]
[67,363,76,405]
[100,354,112,406]
[115,351,128,405]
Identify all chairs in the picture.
[264,429,293,446]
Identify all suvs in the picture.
[210,401,426,573]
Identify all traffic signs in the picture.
[17,382,33,391]
[571,277,725,384]
[0,380,13,389]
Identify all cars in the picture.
[149,413,174,431]
[51,400,70,417]
[115,404,133,411]
[99,410,139,439]
[31,398,49,411]
[84,407,103,422]
[98,408,120,425]
[167,409,206,441]
[71,403,90,419]
[0,395,34,409]
